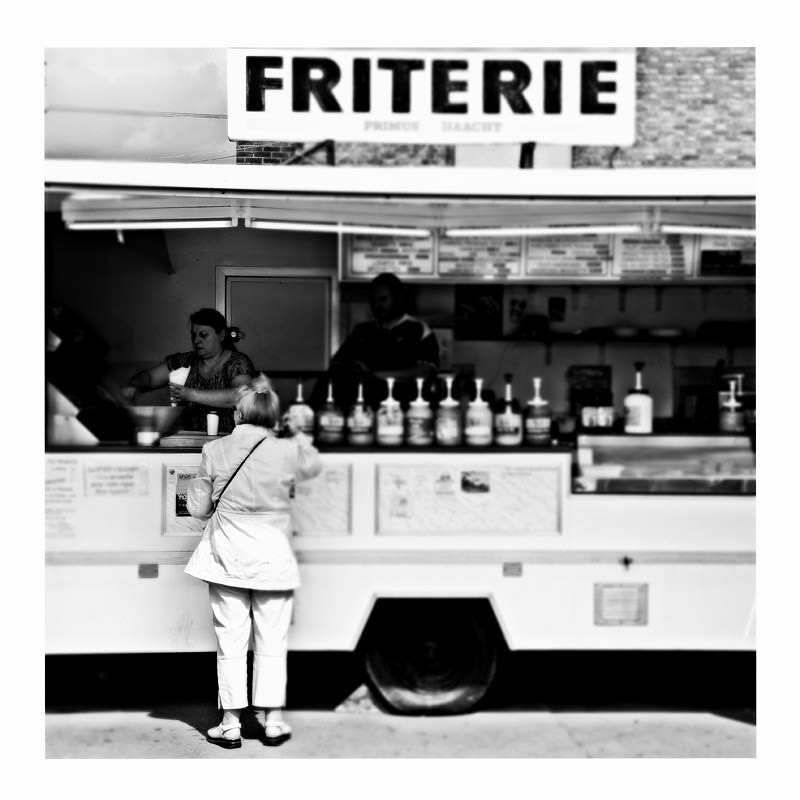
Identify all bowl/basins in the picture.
[123,406,184,445]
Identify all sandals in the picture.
[206,722,242,749]
[265,721,291,745]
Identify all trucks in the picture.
[44,157,755,716]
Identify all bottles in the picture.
[288,387,743,447]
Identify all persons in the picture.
[118,308,255,432]
[185,373,322,748]
[323,272,439,389]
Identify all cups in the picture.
[207,414,219,436]
[137,432,161,446]
